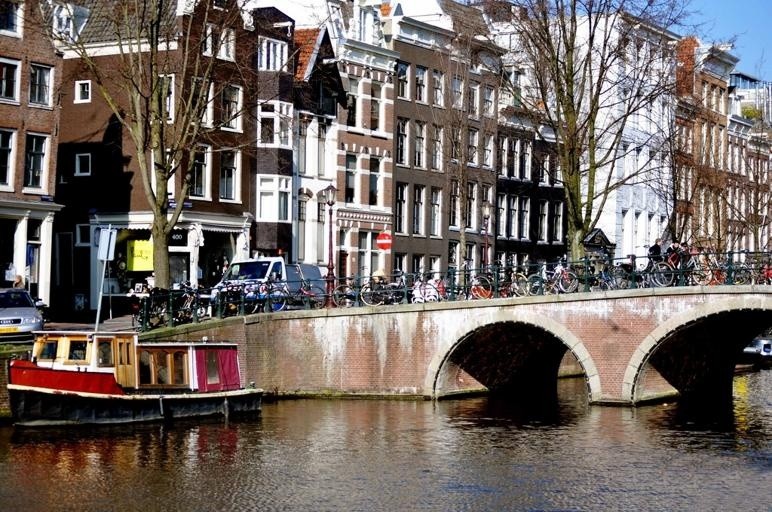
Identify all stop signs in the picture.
[376,233,393,251]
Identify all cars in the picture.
[0,285,46,339]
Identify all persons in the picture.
[649,239,691,286]
[13,275,25,289]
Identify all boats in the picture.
[5,329,262,431]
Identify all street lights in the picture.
[479,200,493,272]
[322,184,341,309]
